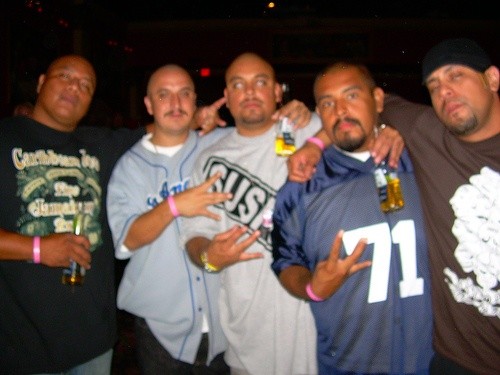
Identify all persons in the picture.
[178,47,405,375]
[286,38,500,375]
[0,55,227,375]
[105,65,311,375]
[271,59,435,375]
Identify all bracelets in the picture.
[200,249,221,273]
[167,196,179,217]
[32,235,40,264]
[305,284,323,302]
[307,137,326,151]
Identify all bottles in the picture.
[274,85,299,157]
[370,123,405,214]
[60,205,92,286]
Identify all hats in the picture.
[421,37,492,84]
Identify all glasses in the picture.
[45,72,94,96]
